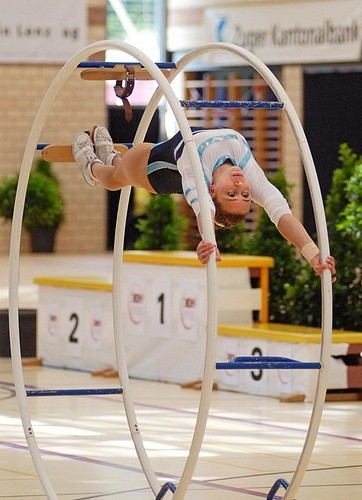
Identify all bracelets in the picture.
[300,241,319,262]
[200,232,217,245]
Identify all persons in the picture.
[72,124,339,283]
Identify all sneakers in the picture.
[71,133,102,188]
[93,127,115,165]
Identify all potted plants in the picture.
[0,156,66,253]
